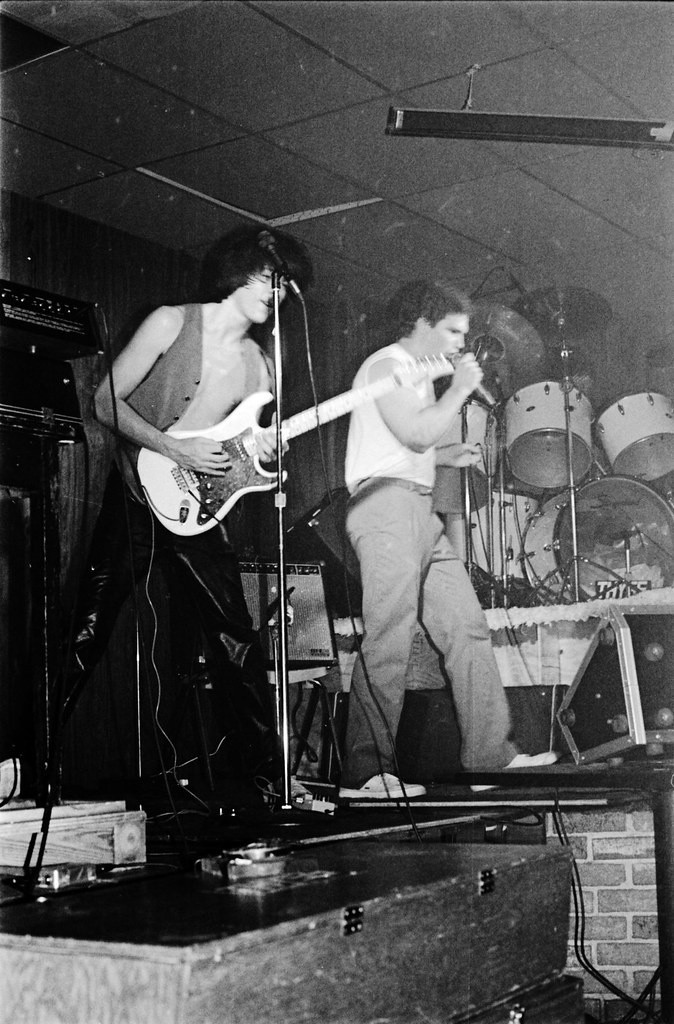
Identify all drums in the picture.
[446,491,537,594]
[520,476,674,604]
[503,380,593,487]
[599,389,674,483]
[433,399,497,514]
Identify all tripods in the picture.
[529,390,637,600]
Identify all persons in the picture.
[84,218,339,834]
[337,274,560,802]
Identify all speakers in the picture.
[555,607,673,766]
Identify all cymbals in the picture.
[460,299,547,385]
[513,287,611,348]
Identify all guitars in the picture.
[138,352,454,536]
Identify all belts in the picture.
[359,477,433,495]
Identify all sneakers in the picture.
[339,773,426,798]
[470,750,557,792]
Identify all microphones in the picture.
[451,352,496,407]
[256,230,299,300]
